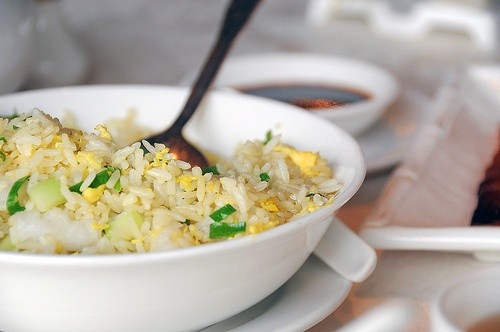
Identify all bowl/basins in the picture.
[1,83,367,332]
[435,263,499,332]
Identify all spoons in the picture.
[137,1,259,168]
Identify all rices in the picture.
[2,106,339,256]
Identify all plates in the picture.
[347,86,431,174]
[360,59,500,261]
[190,255,355,332]
[209,51,397,129]
